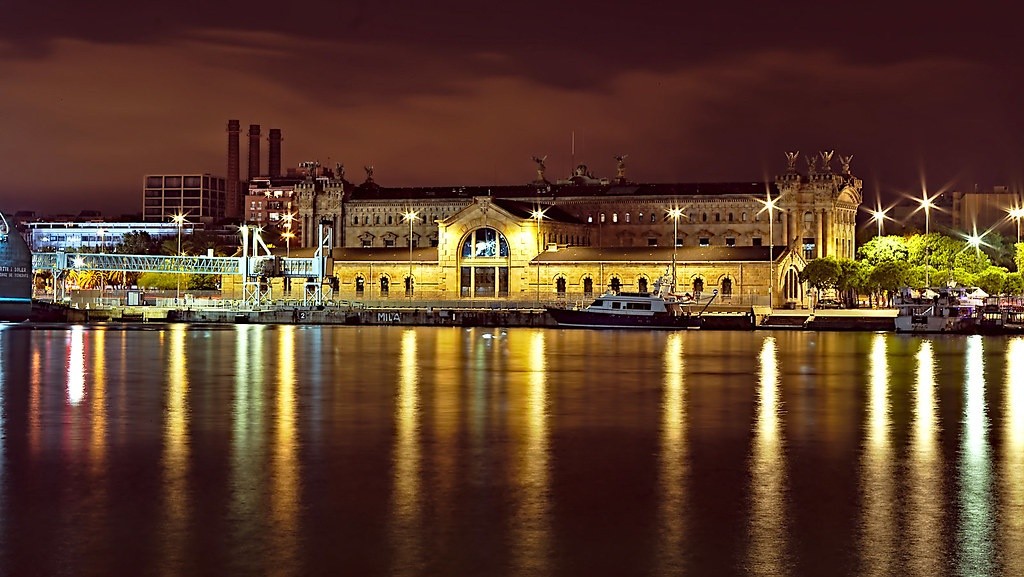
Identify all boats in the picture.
[542,263,719,330]
[892,286,987,334]
[0,210,74,330]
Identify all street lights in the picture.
[409,211,415,303]
[874,211,884,311]
[177,216,183,307]
[673,209,680,292]
[97,228,107,306]
[1013,208,1021,273]
[923,196,931,288]
[536,211,543,304]
[765,199,775,313]
